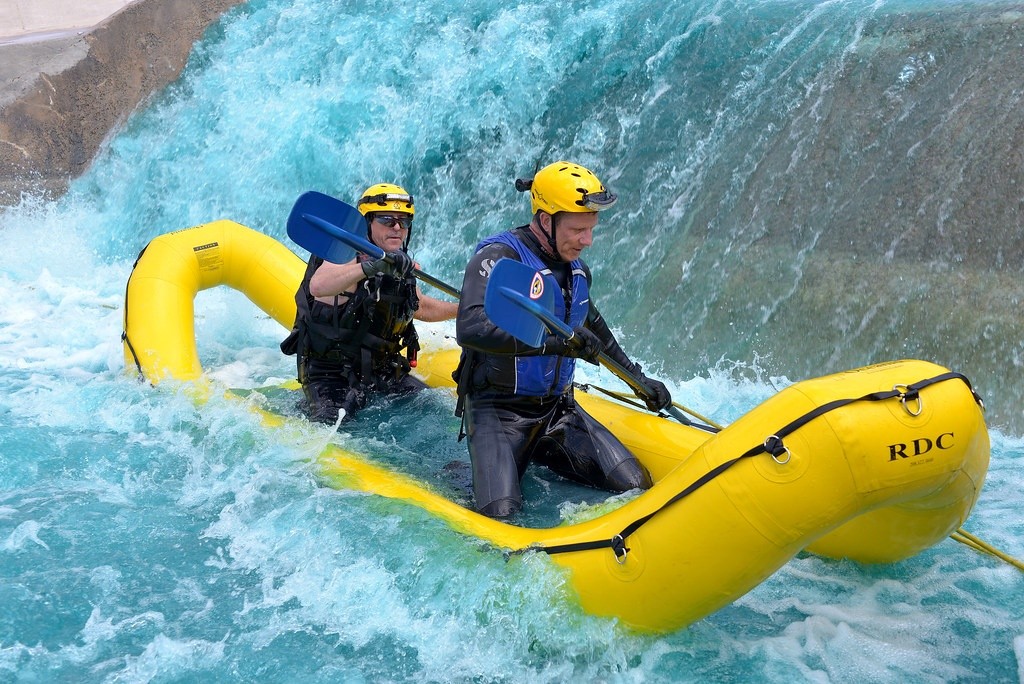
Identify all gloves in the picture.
[630,361,672,413]
[361,250,416,280]
[545,327,600,368]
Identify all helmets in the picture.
[530,161,618,215]
[357,183,415,217]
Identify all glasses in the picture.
[371,214,412,229]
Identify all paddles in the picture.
[286,191,462,301]
[483,255,693,427]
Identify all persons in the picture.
[451,161,671,520]
[280,184,460,415]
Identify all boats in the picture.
[119,220,991,641]
